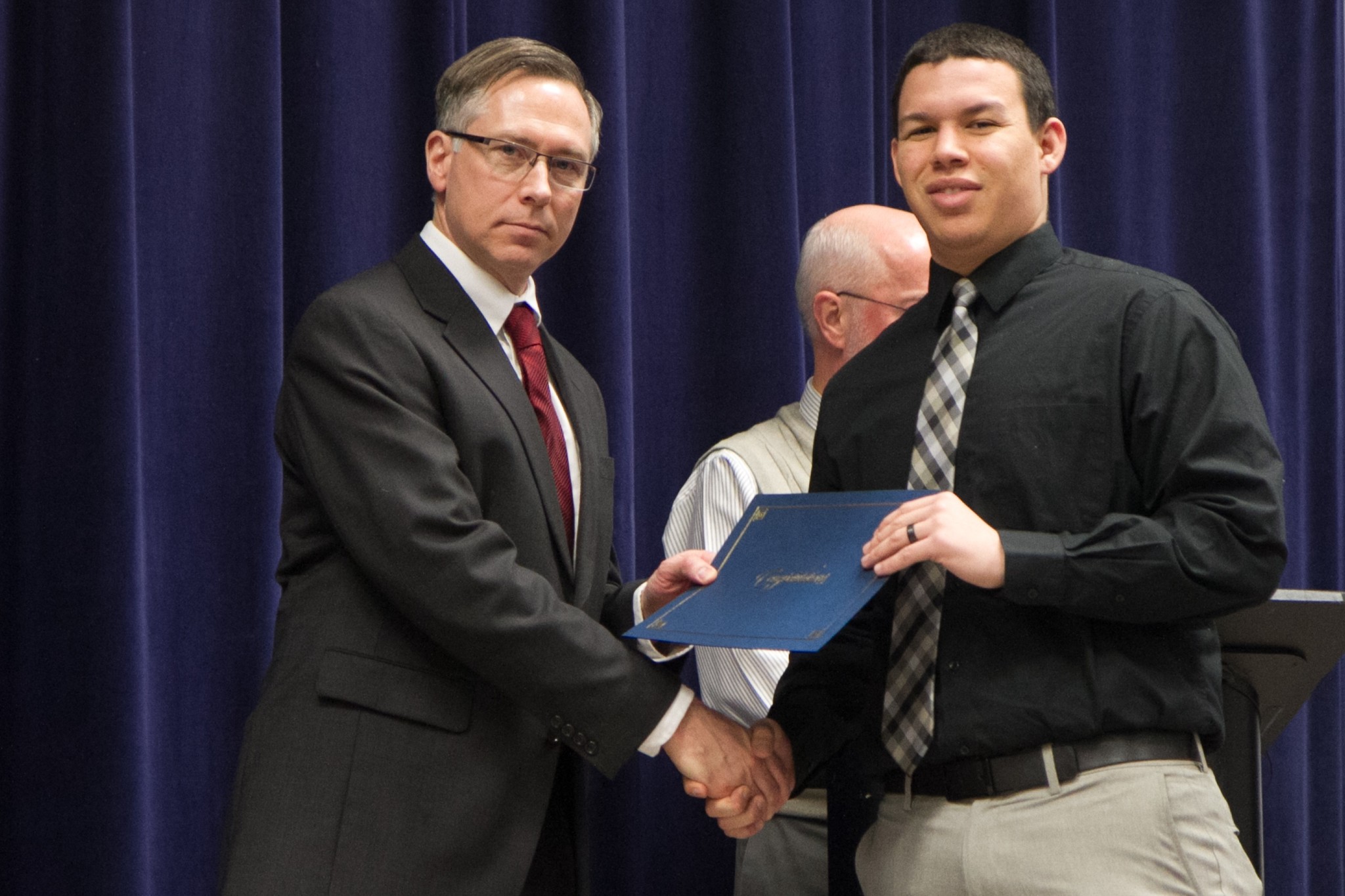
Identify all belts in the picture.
[880,729,1200,801]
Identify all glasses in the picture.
[443,130,596,192]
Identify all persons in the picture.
[661,204,934,896]
[216,36,795,896]
[686,26,1289,896]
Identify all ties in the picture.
[505,298,575,569]
[883,278,985,774]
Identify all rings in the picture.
[907,524,918,543]
